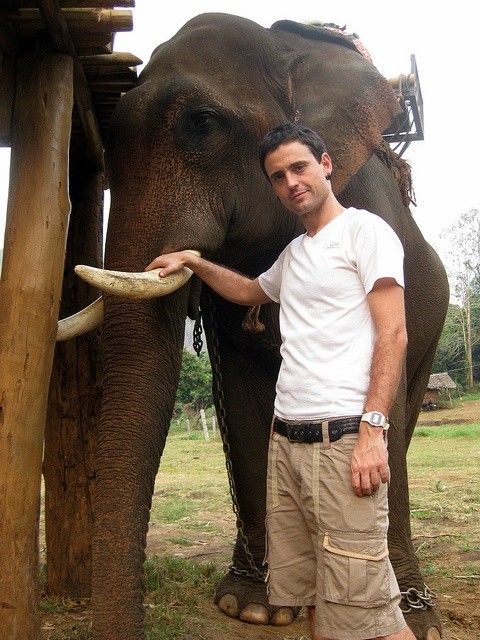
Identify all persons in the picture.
[142,122,417,636]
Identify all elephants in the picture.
[55,12,451,640]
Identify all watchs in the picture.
[362,410,392,432]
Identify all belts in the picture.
[271,416,361,443]
